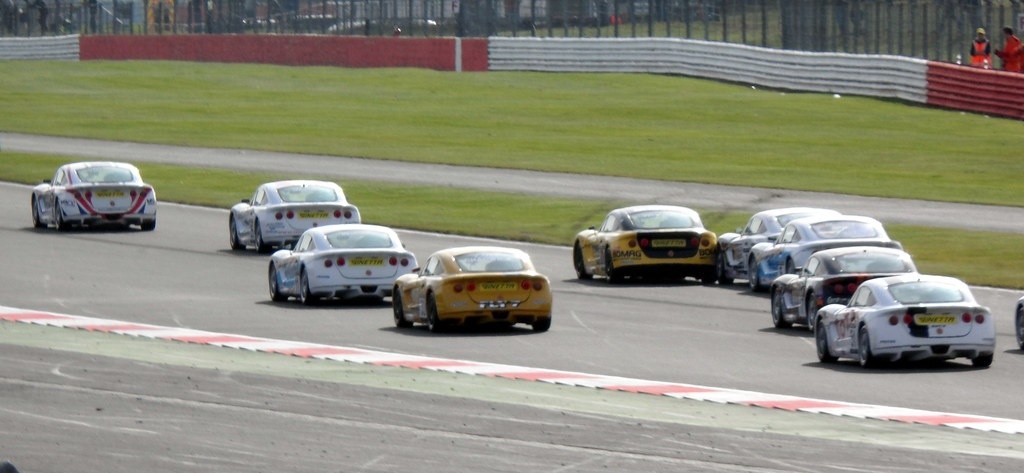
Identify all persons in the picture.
[30,1,50,36]
[968,27,993,71]
[83,0,100,33]
[992,26,1024,76]
[2,0,15,34]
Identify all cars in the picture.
[747,214,904,292]
[32,160,157,232]
[268,223,419,305]
[572,204,718,284]
[229,180,360,253]
[815,273,995,368]
[769,245,919,331]
[719,208,842,284]
[391,245,554,333]
